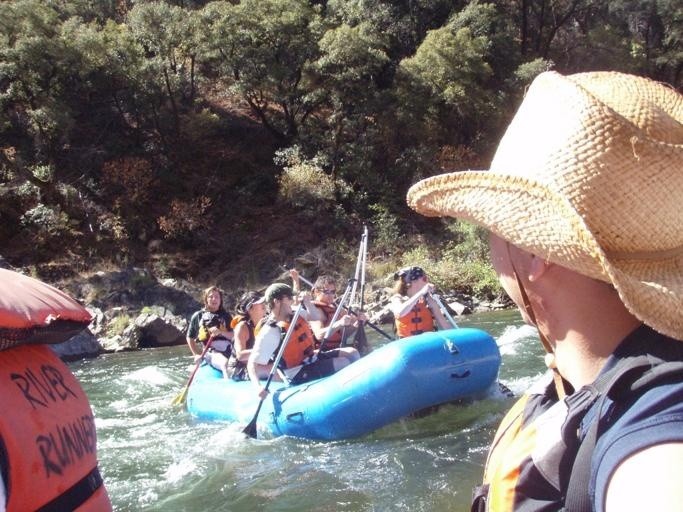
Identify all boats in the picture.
[186,326,501,442]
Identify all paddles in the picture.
[431,292,516,399]
[240,298,306,439]
[170,332,212,407]
[315,222,368,357]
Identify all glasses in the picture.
[322,289,336,295]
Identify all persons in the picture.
[391,266,451,339]
[402,68,683,512]
[0,264,118,511]
[186,268,301,381]
[247,282,361,399]
[307,273,374,363]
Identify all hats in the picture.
[246,294,266,311]
[407,71,682,342]
